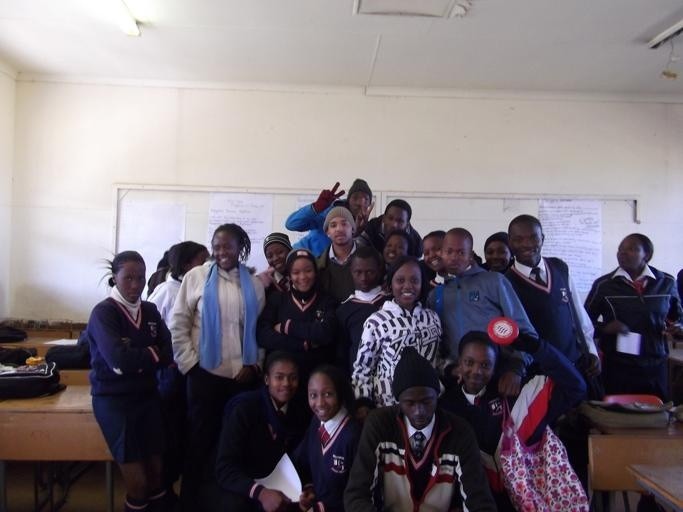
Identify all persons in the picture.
[87,179,600,512]
[585,232,683,406]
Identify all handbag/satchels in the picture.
[499,423,593,512]
[0,326,91,400]
[581,399,673,429]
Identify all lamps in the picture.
[648,19,683,49]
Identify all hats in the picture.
[323,207,356,233]
[348,179,372,204]
[392,346,441,401]
[263,233,293,254]
[286,248,317,273]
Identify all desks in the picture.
[0,327,127,511]
[589,331,683,512]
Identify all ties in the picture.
[632,280,644,294]
[414,432,425,461]
[531,267,546,287]
